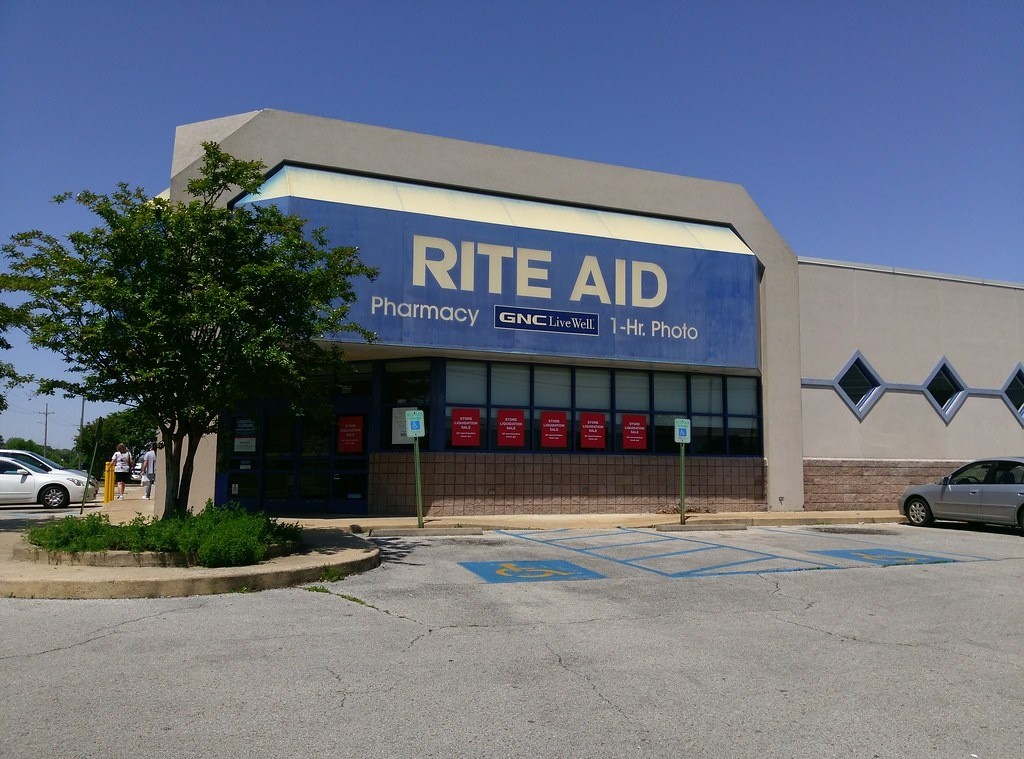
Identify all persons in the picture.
[106,442,135,501]
[141,444,158,500]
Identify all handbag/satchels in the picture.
[140,474,150,487]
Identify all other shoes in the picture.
[142,497,150,500]
[117,495,124,500]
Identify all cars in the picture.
[0,457,97,509]
[898,457,1024,531]
[0,449,99,491]
